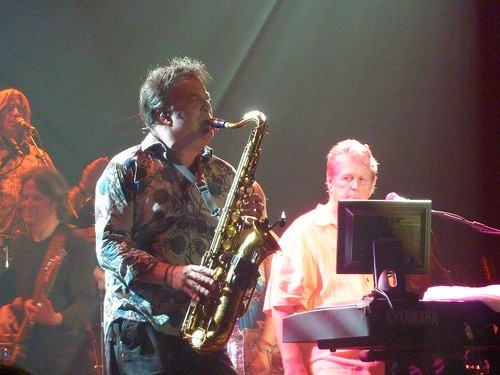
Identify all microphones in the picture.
[10,139,23,155]
[16,117,35,132]
[385,192,408,200]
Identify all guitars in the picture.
[10,249,64,366]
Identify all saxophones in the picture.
[180,109,283,357]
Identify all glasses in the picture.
[4,104,27,116]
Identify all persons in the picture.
[261,139,444,375]
[0,89,112,305]
[220,179,280,375]
[1,169,106,375]
[92,59,267,375]
[66,156,112,228]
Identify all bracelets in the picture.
[76,183,86,196]
[164,264,179,289]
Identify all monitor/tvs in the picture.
[336,199,432,300]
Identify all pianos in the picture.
[281,292,500,375]
